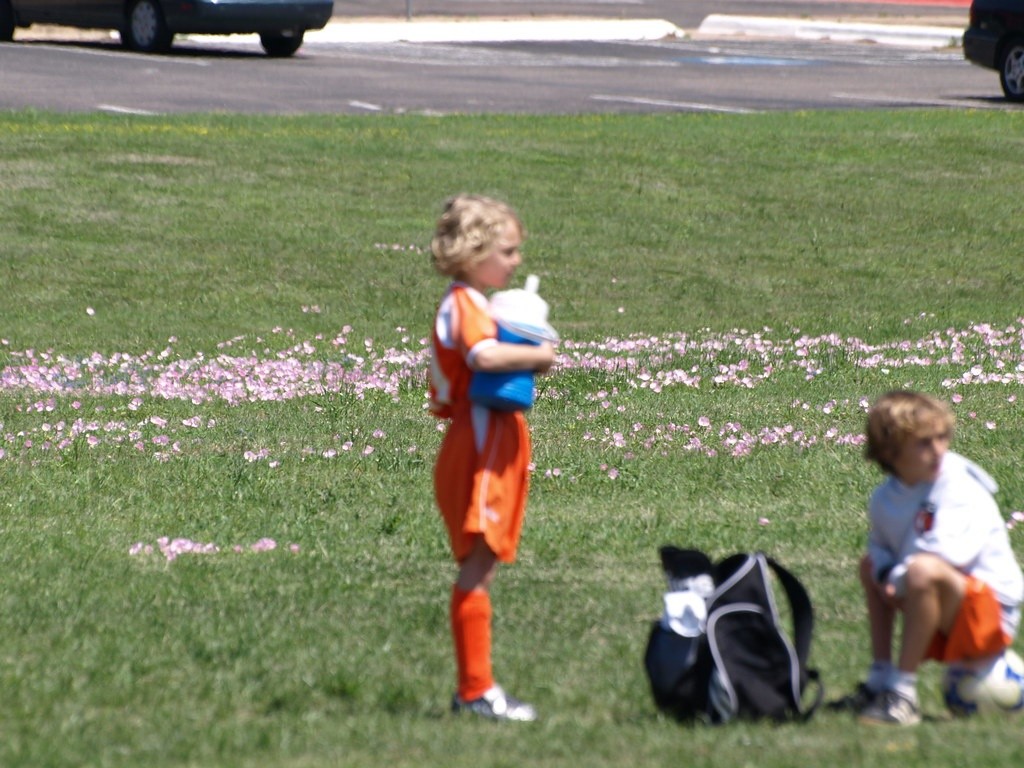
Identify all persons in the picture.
[424,194,557,724]
[821,387,1024,727]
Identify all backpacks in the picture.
[642,545,824,730]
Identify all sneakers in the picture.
[823,682,875,718]
[853,685,924,729]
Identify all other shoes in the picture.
[450,683,537,726]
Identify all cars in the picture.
[0,0,336,58]
[961,0,1024,103]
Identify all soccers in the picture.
[942,646,1024,716]
